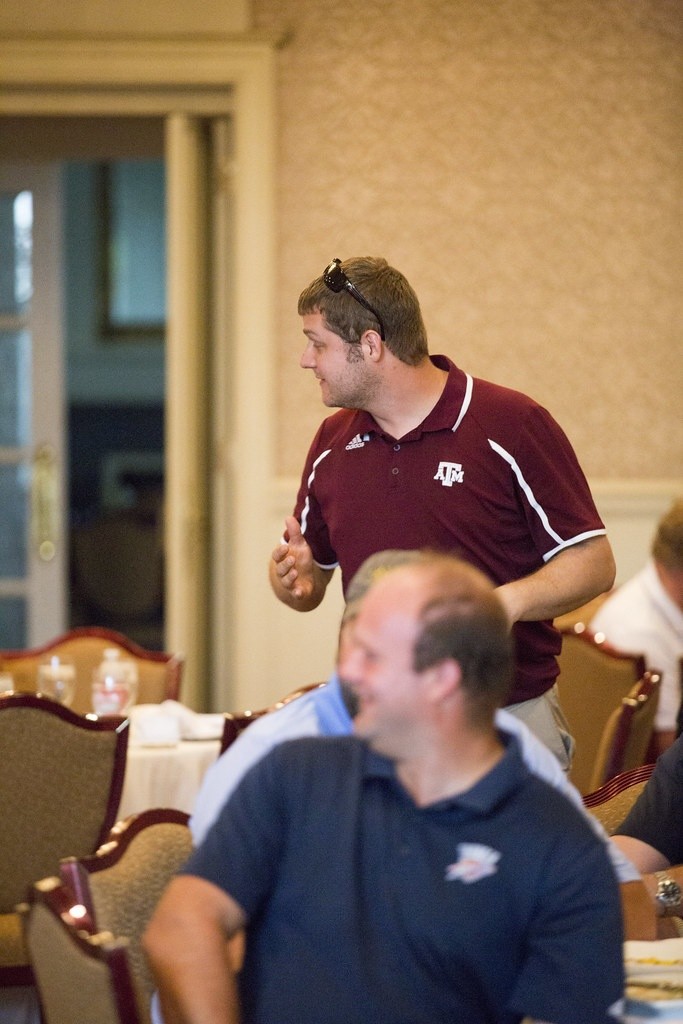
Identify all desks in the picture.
[116,738,222,822]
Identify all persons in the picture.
[591,496,683,918]
[268,256,617,773]
[143,548,654,1024]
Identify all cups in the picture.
[92,659,138,716]
[37,662,77,708]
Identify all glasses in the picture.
[323,257,386,340]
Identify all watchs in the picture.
[654,870,683,917]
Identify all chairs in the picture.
[14,872,145,1024]
[56,808,194,1024]
[0,689,130,1024]
[587,667,664,792]
[580,763,659,840]
[0,625,186,714]
[554,623,649,794]
